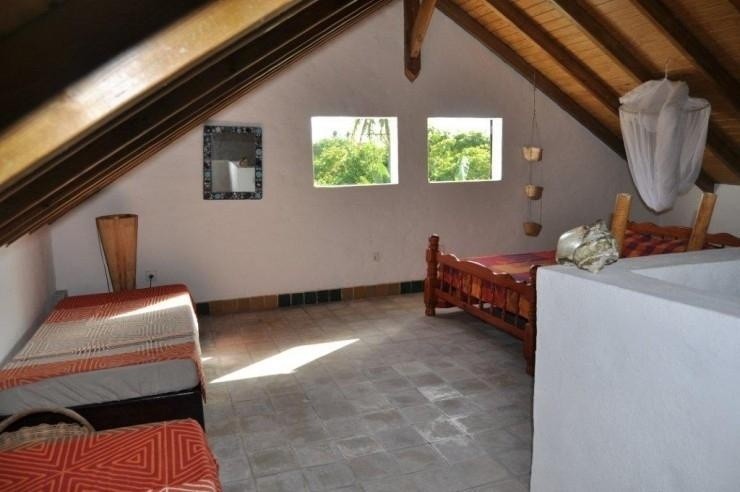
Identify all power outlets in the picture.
[144,270,157,283]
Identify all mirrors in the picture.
[202,122,264,200]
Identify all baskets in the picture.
[0,404,96,450]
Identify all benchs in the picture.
[0,406,225,492]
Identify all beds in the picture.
[1,282,209,434]
[424,212,740,376]
